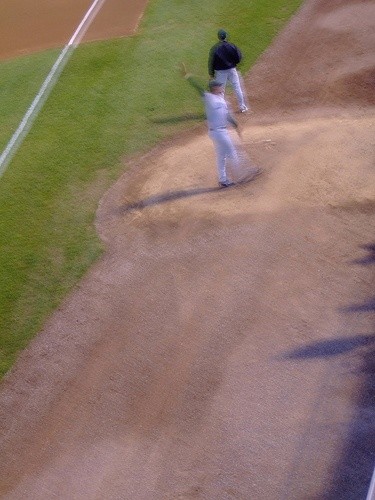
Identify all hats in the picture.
[209,79,225,87]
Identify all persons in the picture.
[208,29,249,114]
[177,60,262,187]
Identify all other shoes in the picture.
[241,106,248,112]
